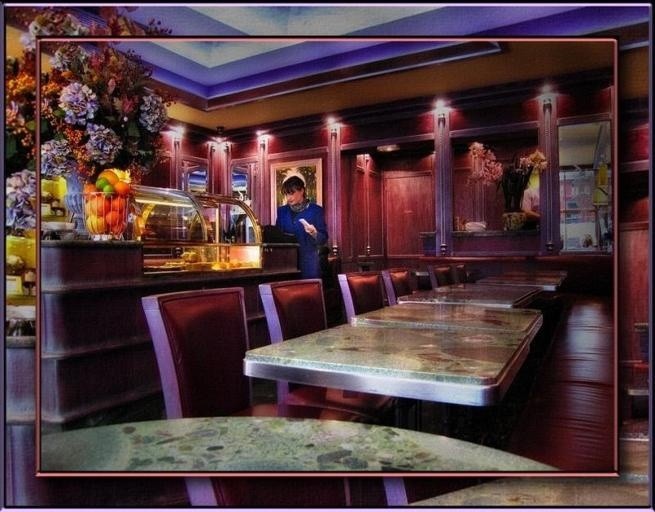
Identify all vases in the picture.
[63,177,86,237]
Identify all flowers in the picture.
[5,4,176,228]
[40,43,178,234]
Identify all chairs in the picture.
[140,284,363,506]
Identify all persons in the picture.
[521,188,542,219]
[273,175,328,279]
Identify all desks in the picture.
[242,265,570,424]
[409,413,652,506]
[41,416,560,472]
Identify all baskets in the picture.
[80,192,131,240]
